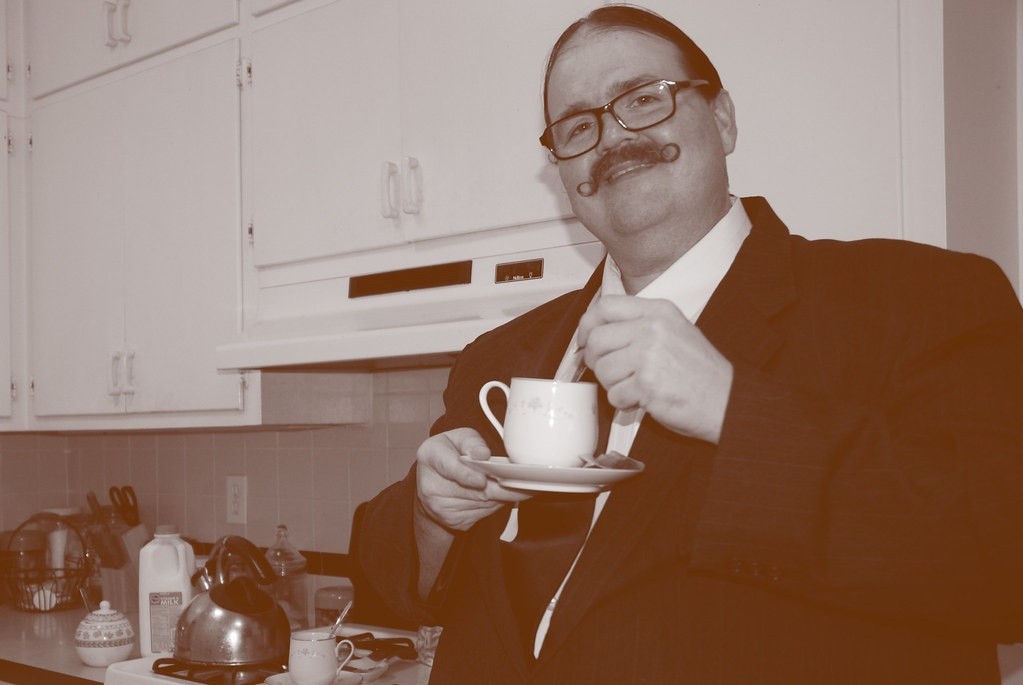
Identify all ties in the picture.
[504,367,617,658]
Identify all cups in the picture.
[1,530,47,598]
[479,376,599,466]
[315,586,356,626]
[288,632,355,684]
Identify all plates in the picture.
[263,667,363,684]
[339,656,389,683]
[458,454,646,493]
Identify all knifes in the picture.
[86,489,124,568]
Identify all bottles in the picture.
[138,524,197,657]
[265,524,309,632]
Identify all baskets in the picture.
[0,512,90,613]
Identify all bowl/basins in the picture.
[72,600,134,667]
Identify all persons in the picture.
[345,5,1023,685]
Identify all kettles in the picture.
[174,534,291,666]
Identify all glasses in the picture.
[538,77,713,161]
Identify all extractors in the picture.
[213,219,612,374]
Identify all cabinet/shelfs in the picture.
[0,1,948,431]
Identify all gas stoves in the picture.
[122,623,426,685]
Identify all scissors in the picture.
[107,484,141,527]
